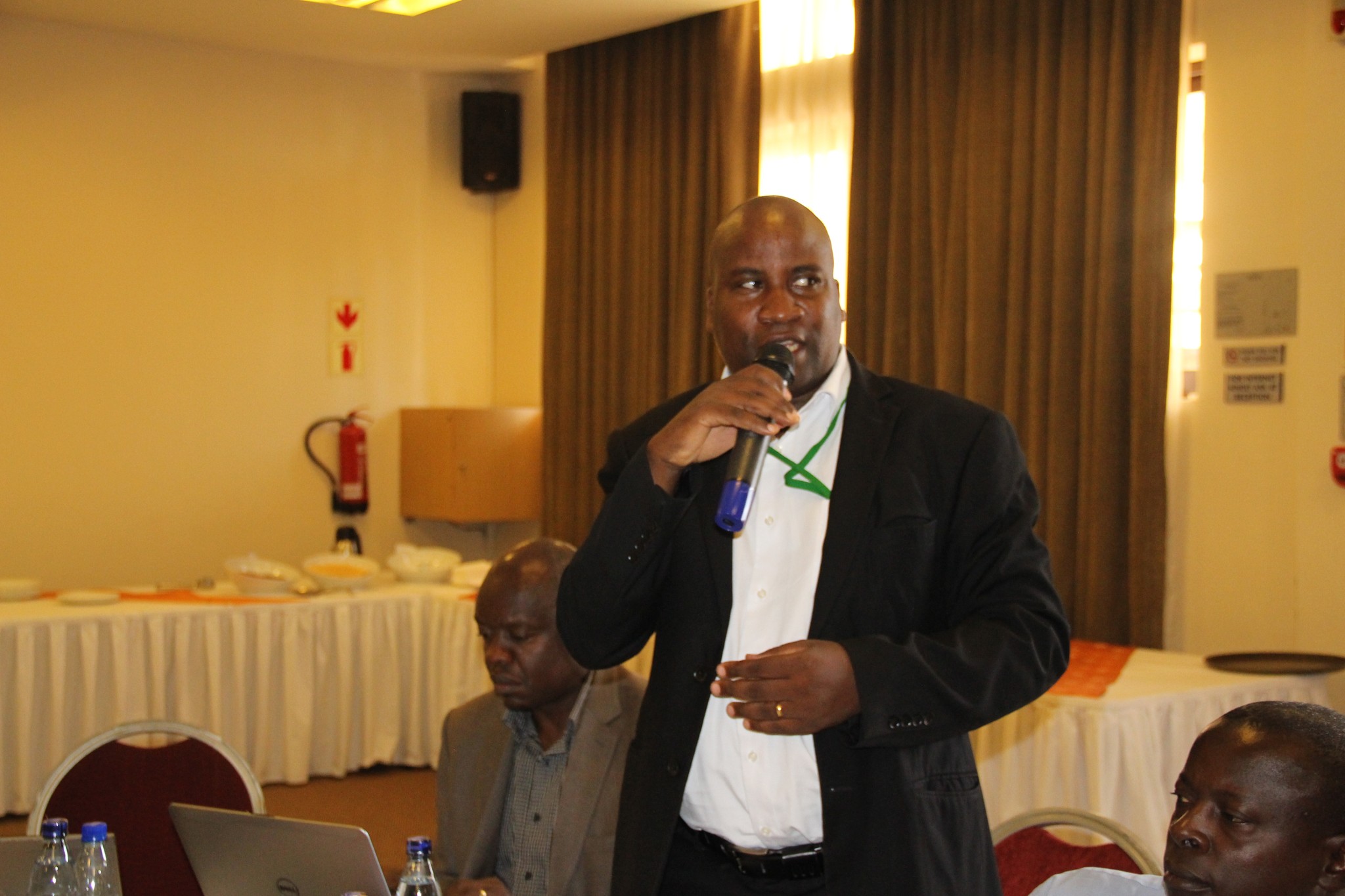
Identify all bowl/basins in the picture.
[303,554,381,588]
[386,545,460,582]
[232,571,288,593]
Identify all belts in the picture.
[687,825,824,879]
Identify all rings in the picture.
[479,890,487,896]
[776,702,782,717]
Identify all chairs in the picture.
[26,720,266,896]
[990,805,1165,896]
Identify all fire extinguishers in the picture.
[304,408,373,515]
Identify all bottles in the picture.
[395,837,442,896]
[65,822,117,896]
[26,818,72,896]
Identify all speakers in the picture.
[461,90,521,192]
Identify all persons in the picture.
[1027,700,1345,896]
[384,537,645,896]
[555,197,1070,896]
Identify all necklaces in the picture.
[766,386,851,500]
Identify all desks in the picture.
[0,571,1345,870]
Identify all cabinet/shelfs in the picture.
[399,410,544,521]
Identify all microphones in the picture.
[714,344,795,532]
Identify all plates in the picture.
[58,592,119,605]
